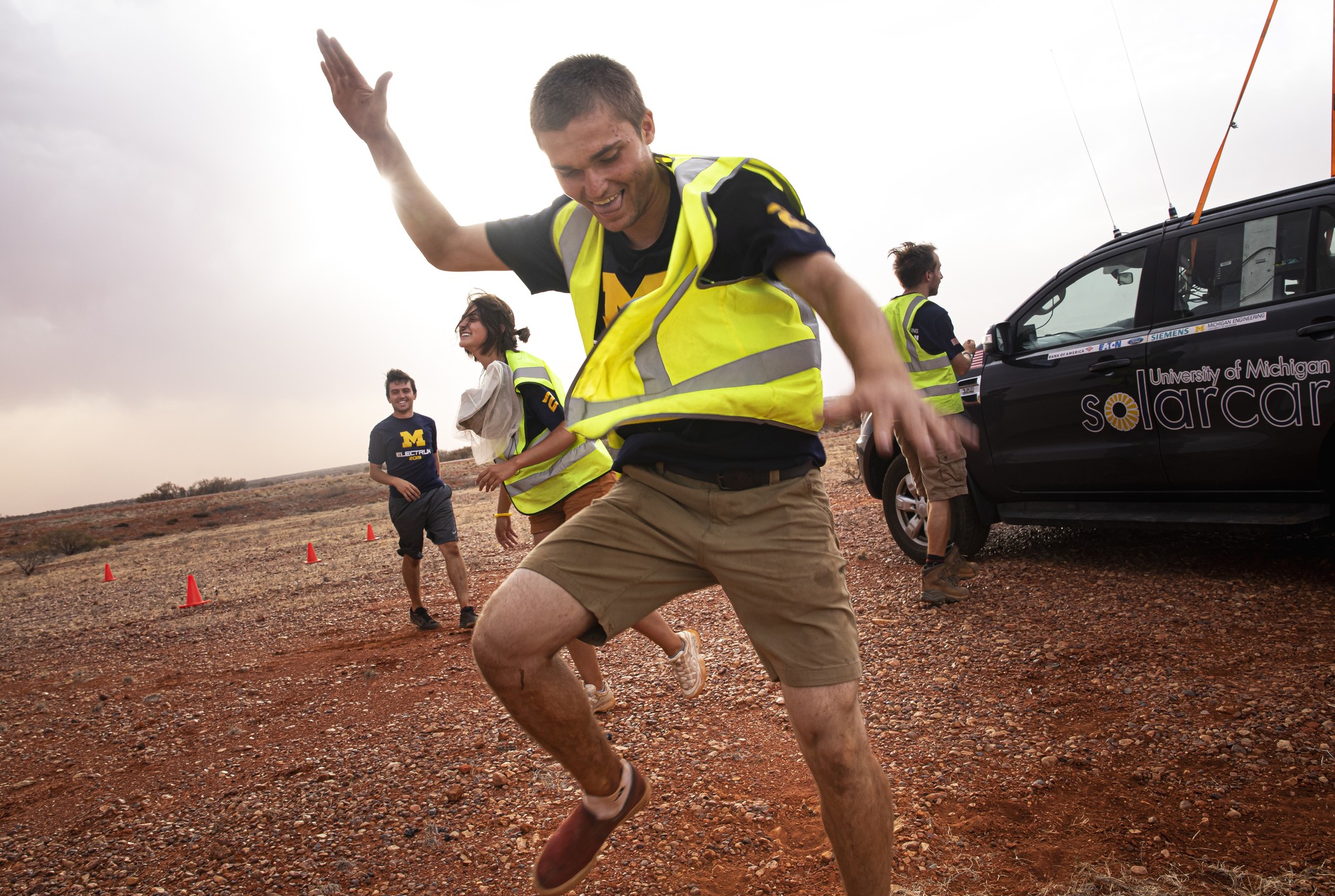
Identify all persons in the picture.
[313,28,978,895]
[368,369,479,629]
[453,293,706,714]
[875,241,980,605]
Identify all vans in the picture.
[857,173,1335,566]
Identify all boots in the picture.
[944,543,979,586]
[920,563,971,602]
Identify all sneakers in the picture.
[579,680,616,713]
[667,629,706,699]
[410,606,443,629]
[533,764,652,896]
[459,606,479,628]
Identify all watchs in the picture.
[963,352,973,363]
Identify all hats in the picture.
[456,361,521,465]
